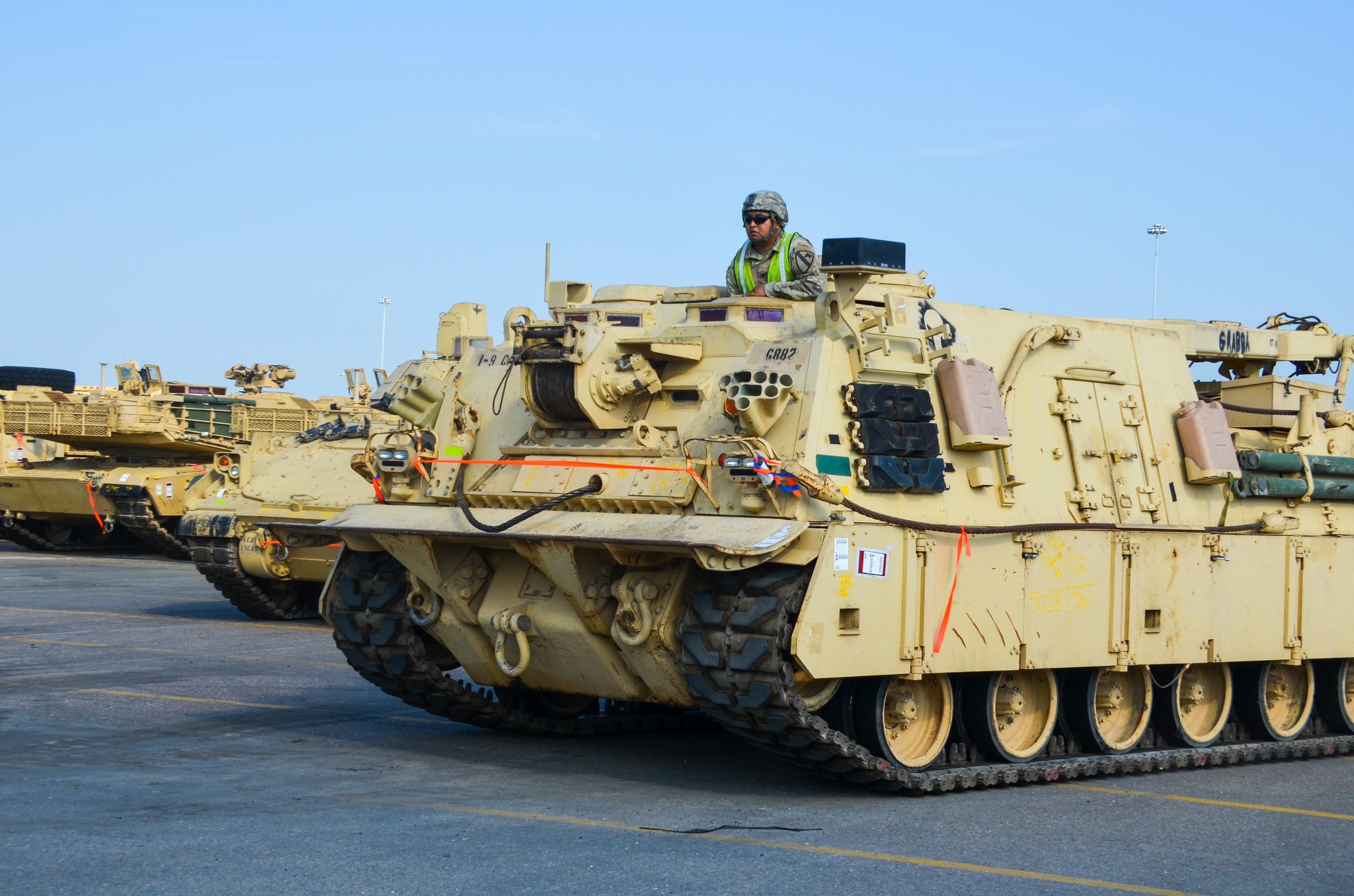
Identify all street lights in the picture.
[1146,223,1168,320]
[379,296,392,369]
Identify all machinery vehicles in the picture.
[329,236,1353,793]
[1,355,403,624]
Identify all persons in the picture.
[724,191,824,321]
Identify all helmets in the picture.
[742,191,789,222]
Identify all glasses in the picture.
[744,215,771,224]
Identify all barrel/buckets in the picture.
[936,356,1013,451]
[1177,400,1242,484]
